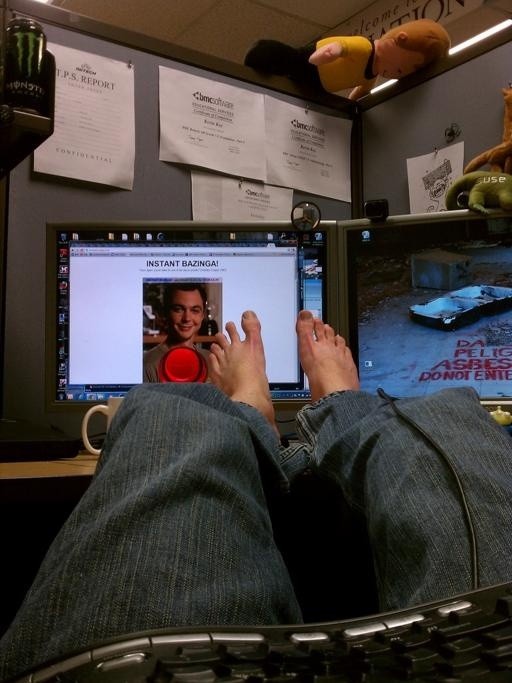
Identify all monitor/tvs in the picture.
[45,220,339,415]
[340,208,512,411]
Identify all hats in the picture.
[156,345,209,384]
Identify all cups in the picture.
[3,17,51,114]
[80,396,126,456]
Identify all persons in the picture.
[243,17,450,100]
[1,308,512,683]
[142,283,213,384]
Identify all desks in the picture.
[2,449,100,484]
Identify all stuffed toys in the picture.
[443,170,512,216]
